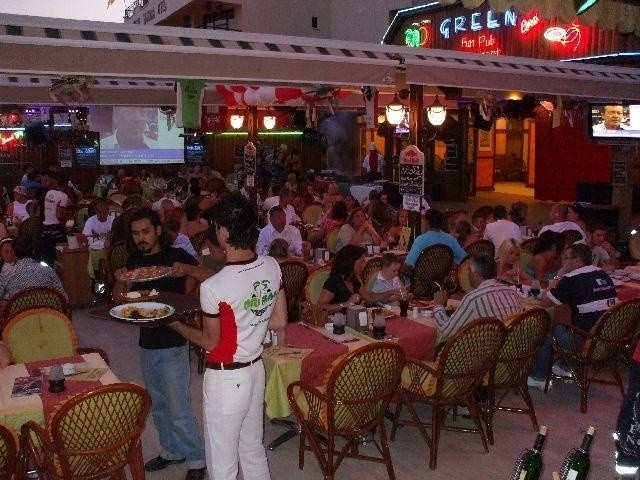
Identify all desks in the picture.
[262,263,637,452]
[0,351,126,447]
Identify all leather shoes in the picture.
[444,404,486,419]
[186,468,206,480]
[145,455,186,472]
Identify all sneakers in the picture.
[526,375,554,393]
[551,364,574,384]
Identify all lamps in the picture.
[384,93,406,129]
[427,94,447,127]
[228,105,245,130]
[263,106,276,130]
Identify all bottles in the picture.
[515,424,548,479]
[510,271,522,292]
[413,307,419,319]
[47,365,68,394]
[369,313,388,338]
[272,330,280,351]
[331,311,348,336]
[559,426,597,480]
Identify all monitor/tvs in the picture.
[589,101,640,140]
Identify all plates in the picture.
[109,301,175,323]
[606,268,640,288]
[409,300,434,309]
[120,266,170,283]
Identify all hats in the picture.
[369,142,377,151]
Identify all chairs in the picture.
[2,173,640,366]
[21,380,153,480]
[545,294,639,414]
[286,338,405,480]
[2,424,23,480]
[462,303,553,444]
[391,314,508,471]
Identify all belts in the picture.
[205,354,262,371]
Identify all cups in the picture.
[400,299,407,317]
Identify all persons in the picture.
[128,207,217,480]
[593,103,630,131]
[0,144,640,418]
[102,107,161,150]
[167,188,287,480]
